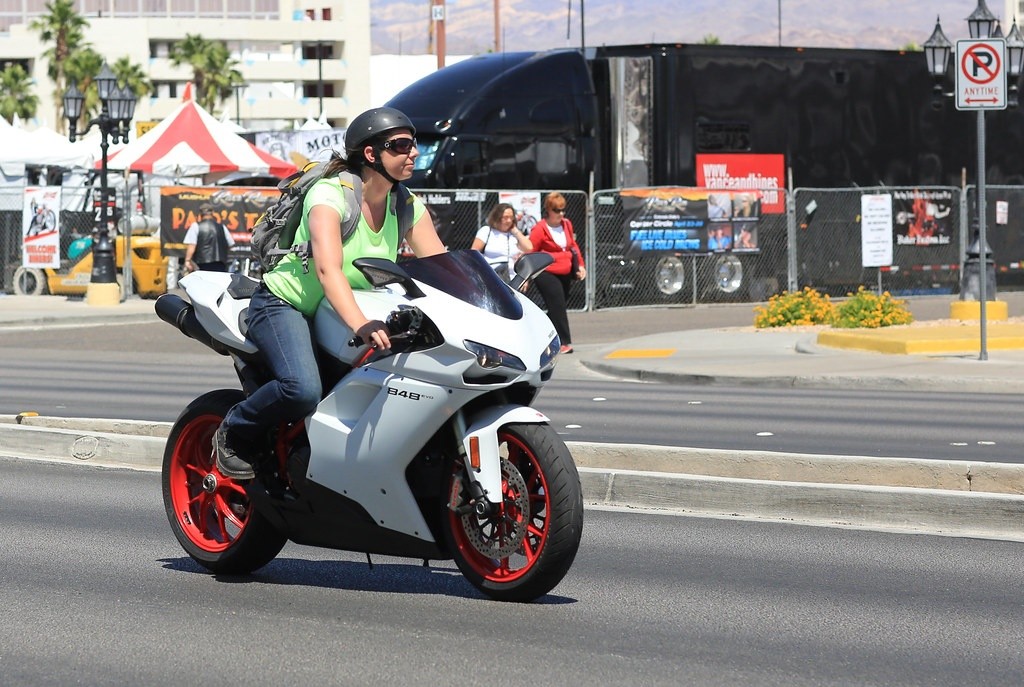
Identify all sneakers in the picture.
[561,345,573,354]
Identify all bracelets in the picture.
[185,260,191,262]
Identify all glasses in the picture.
[552,208,566,214]
[502,216,514,221]
[376,138,417,154]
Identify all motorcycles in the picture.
[153,248,585,602]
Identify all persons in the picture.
[180,201,235,273]
[304,10,312,20]
[26,198,40,236]
[471,192,587,354]
[212,111,446,479]
[708,192,757,250]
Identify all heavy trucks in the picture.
[380,41,1024,304]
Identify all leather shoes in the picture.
[212,428,255,479]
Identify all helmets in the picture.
[345,107,416,154]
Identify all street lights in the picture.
[920,0,1024,361]
[64,57,138,307]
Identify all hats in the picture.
[200,203,212,209]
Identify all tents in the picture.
[0,111,129,219]
[91,98,297,297]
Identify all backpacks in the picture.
[249,161,414,272]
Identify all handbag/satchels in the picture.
[571,248,579,281]
[493,263,510,285]
